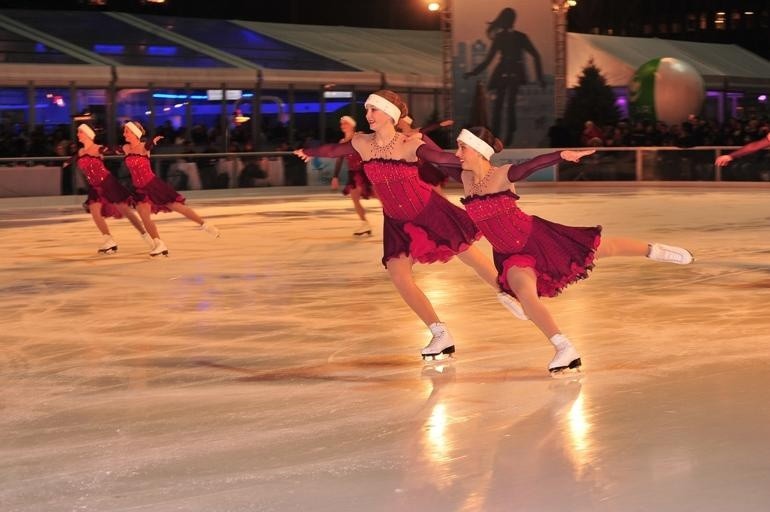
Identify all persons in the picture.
[109,121,221,255]
[331,113,453,235]
[1,110,90,194]
[142,122,308,190]
[290,89,528,355]
[62,122,168,256]
[404,124,693,374]
[581,113,770,181]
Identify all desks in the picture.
[1,161,88,197]
[167,148,288,191]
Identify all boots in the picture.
[150,238,170,256]
[200,221,218,237]
[141,231,152,247]
[647,241,693,265]
[548,333,581,373]
[421,322,455,357]
[98,234,118,252]
[497,291,531,320]
[353,219,372,235]
